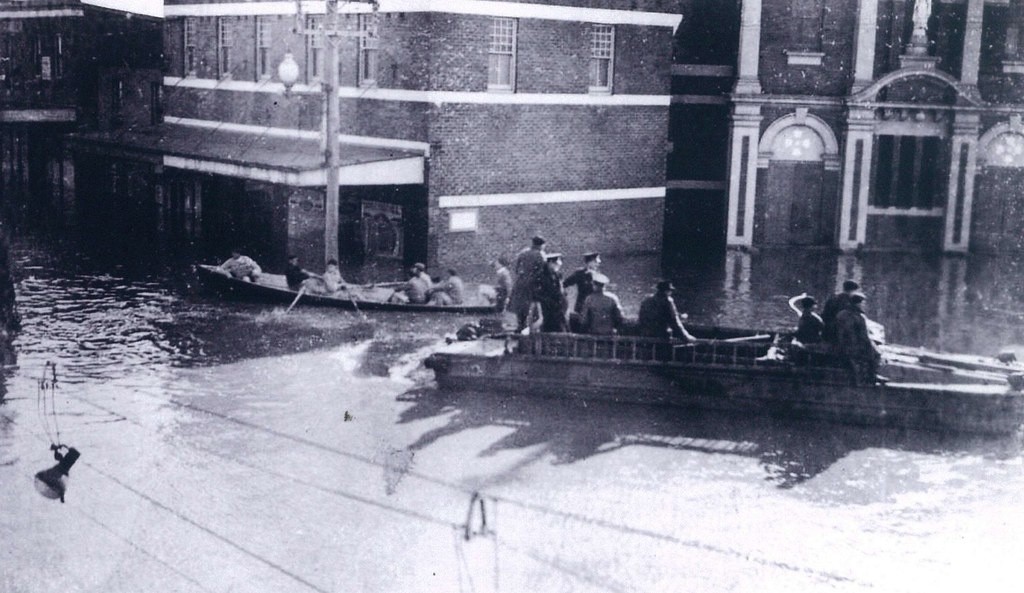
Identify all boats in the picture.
[423,313,1024,436]
[197,263,499,313]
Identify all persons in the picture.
[285,253,324,294]
[220,250,261,282]
[490,256,513,315]
[508,236,568,332]
[636,281,697,342]
[799,281,890,385]
[562,252,625,336]
[318,259,362,300]
[387,263,464,307]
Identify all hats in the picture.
[849,294,864,306]
[655,280,675,290]
[801,296,816,311]
[532,236,547,245]
[843,281,859,290]
[591,272,610,287]
[582,251,601,264]
[546,253,563,265]
